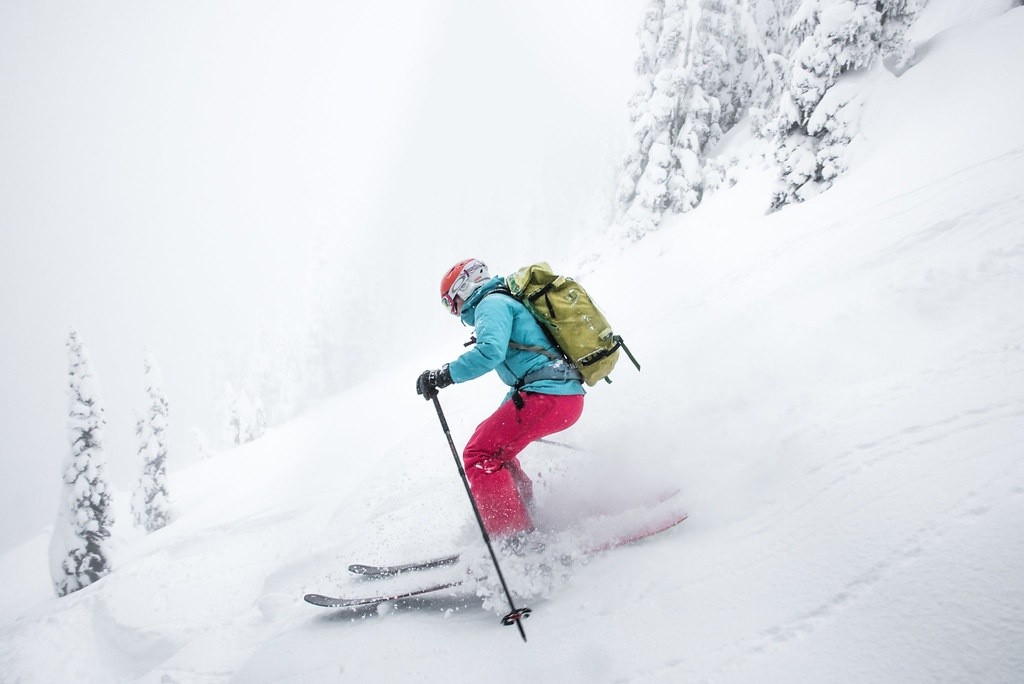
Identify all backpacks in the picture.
[475,262,640,387]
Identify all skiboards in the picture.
[346,483,682,576]
[303,510,689,609]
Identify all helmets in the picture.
[440,258,491,300]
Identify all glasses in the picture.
[440,261,485,314]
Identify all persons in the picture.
[416,258,641,569]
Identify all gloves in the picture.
[416,363,454,401]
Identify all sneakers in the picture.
[491,528,546,557]
